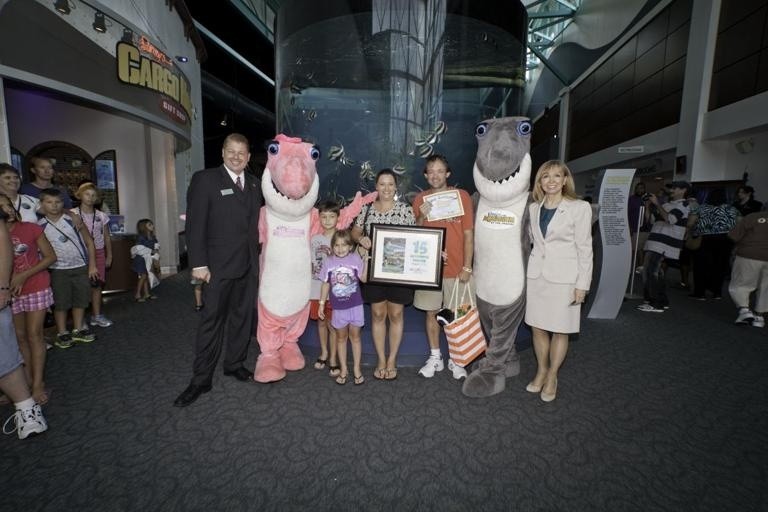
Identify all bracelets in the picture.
[462,266,472,273]
[318,300,325,305]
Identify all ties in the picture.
[236,177,242,190]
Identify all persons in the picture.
[190,278,205,312]
[351,167,416,381]
[310,201,364,377]
[317,230,369,385]
[173,135,266,409]
[0,156,113,440]
[130,219,160,303]
[625,181,768,329]
[412,154,474,380]
[523,160,593,403]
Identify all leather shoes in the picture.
[224,366,253,382]
[527,374,557,401]
[174,378,213,407]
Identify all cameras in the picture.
[642,193,652,201]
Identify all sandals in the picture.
[132,294,157,303]
[194,302,204,311]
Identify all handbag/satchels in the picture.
[443,305,486,366]
[353,244,368,283]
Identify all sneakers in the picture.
[734,311,765,329]
[91,312,112,328]
[14,403,48,439]
[73,328,95,343]
[419,354,444,378]
[54,332,73,349]
[637,301,670,313]
[448,358,467,381]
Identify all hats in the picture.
[666,180,691,188]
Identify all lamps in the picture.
[175,56,188,64]
[52,1,138,48]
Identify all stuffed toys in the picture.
[408,116,603,398]
[252,134,380,383]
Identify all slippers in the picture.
[314,354,349,384]
[375,365,398,379]
[353,371,364,384]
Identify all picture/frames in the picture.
[367,223,447,291]
